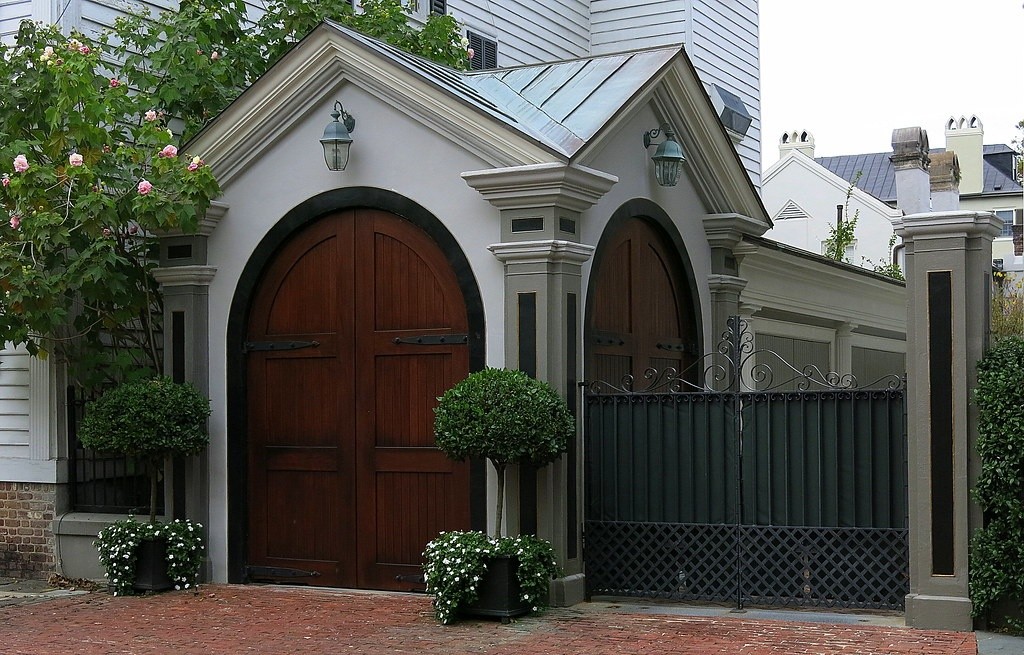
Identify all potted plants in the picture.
[76,374,214,595]
[422,364,575,625]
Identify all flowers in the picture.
[92,510,205,596]
[422,529,568,625]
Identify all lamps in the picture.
[318,100,356,171]
[643,123,686,187]
